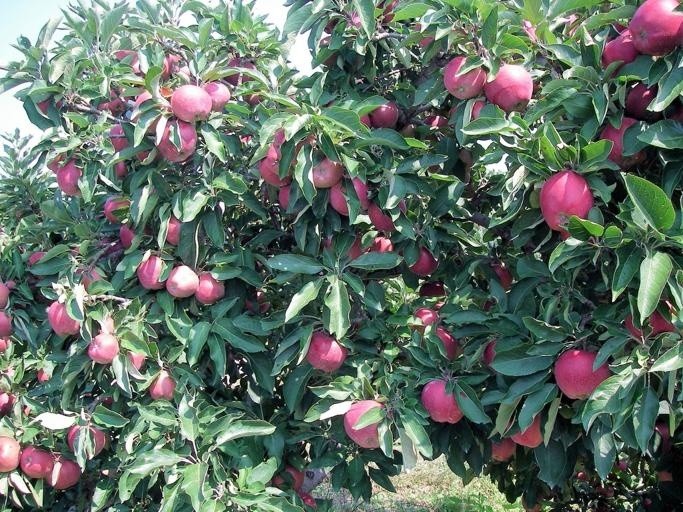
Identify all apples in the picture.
[0,1,681,512]
